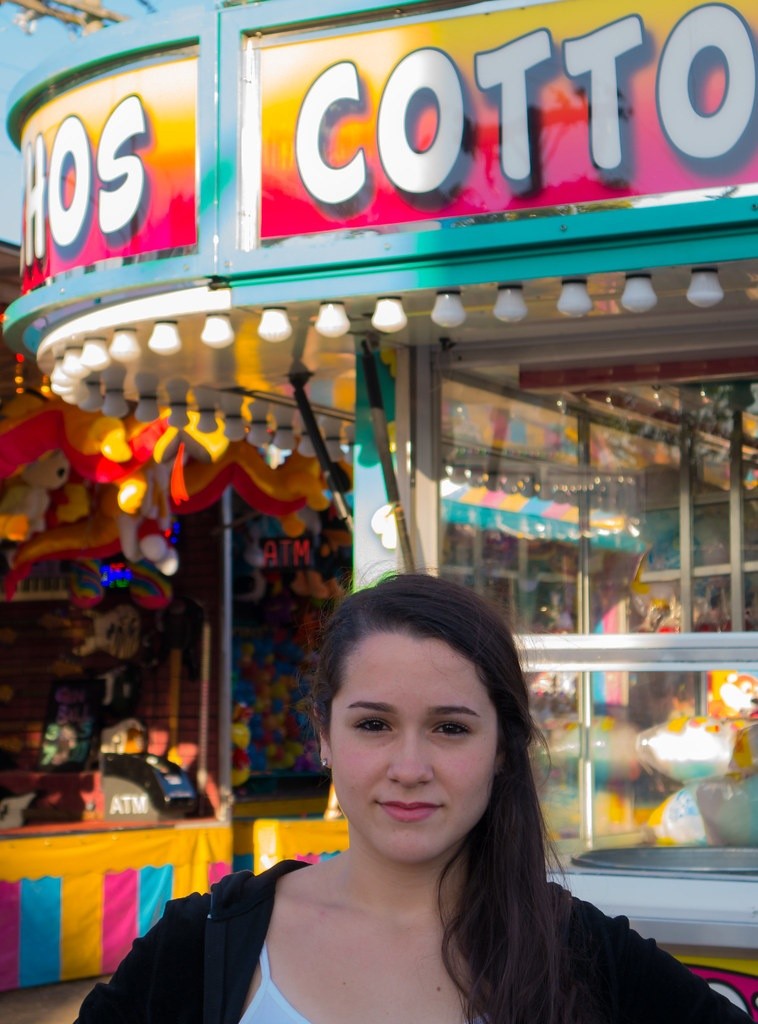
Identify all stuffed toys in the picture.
[0,439,179,576]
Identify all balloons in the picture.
[226,633,347,787]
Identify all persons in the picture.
[68,568,758,1024]
